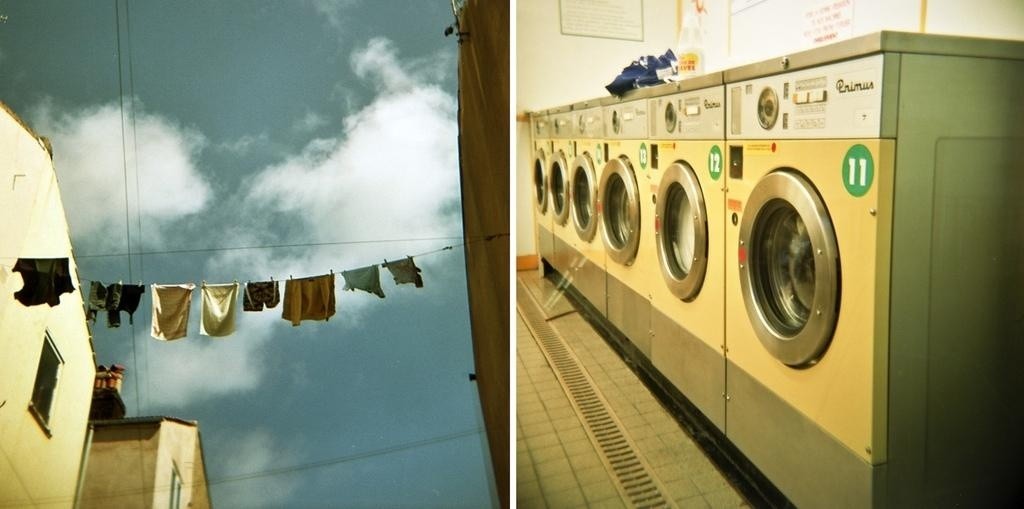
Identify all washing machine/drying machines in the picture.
[521,29,1024,509]
